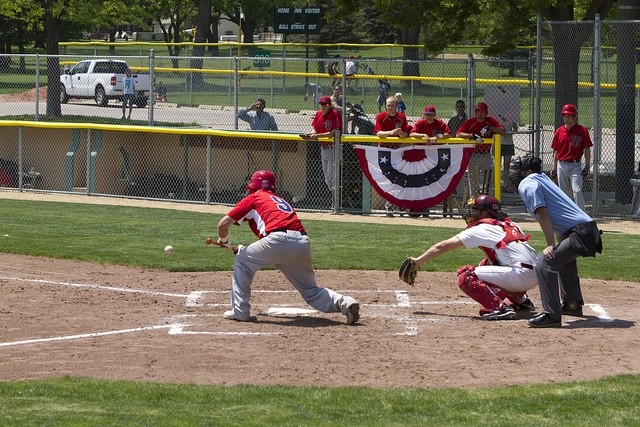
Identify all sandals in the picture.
[502,186,513,194]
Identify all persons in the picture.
[409,106,452,144]
[492,121,516,194]
[374,96,412,217]
[327,54,343,88]
[331,84,356,134]
[410,195,540,321]
[345,56,360,88]
[217,169,360,325]
[156,81,168,101]
[306,96,340,210]
[448,100,468,137]
[456,102,505,198]
[395,93,406,116]
[362,62,376,75]
[236,98,278,131]
[510,156,600,328]
[121,68,137,120]
[303,81,324,106]
[549,103,593,213]
[375,75,391,113]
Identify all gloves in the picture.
[217,237,232,251]
[478,126,492,137]
[550,169,558,180]
[582,165,590,176]
[469,133,482,145]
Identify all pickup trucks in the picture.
[60,59,156,107]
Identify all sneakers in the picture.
[480,305,517,321]
[514,297,535,312]
[344,298,360,325]
[223,309,249,321]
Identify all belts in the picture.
[560,159,581,164]
[272,229,307,236]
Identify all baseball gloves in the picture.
[399,258,417,285]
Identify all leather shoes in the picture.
[527,313,562,329]
[560,303,583,316]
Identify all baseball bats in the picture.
[474,164,480,195]
[443,199,447,217]
[449,198,454,217]
[462,169,469,210]
[486,169,492,193]
[205,237,238,253]
[482,169,487,194]
[451,192,465,216]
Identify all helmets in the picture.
[560,104,577,120]
[245,169,277,192]
[509,155,542,194]
[463,195,479,225]
[472,193,503,219]
[474,102,488,116]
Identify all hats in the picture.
[423,105,436,115]
[318,95,331,104]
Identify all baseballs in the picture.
[164,246,174,255]
